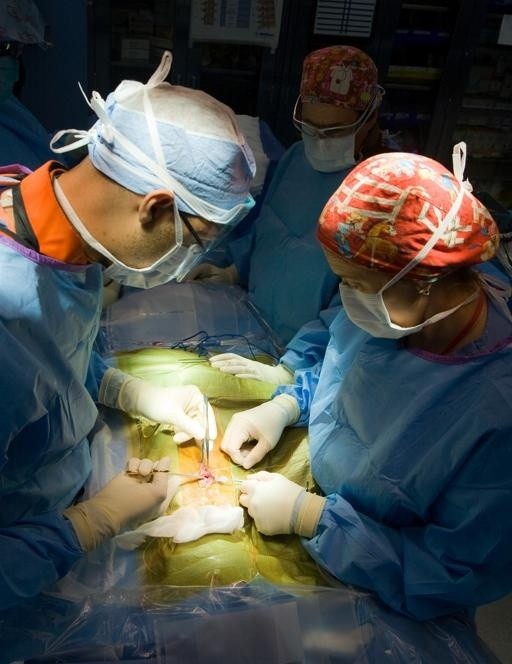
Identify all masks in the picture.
[337,267,437,340]
[89,197,196,291]
[294,112,371,173]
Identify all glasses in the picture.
[291,95,376,141]
[179,211,214,257]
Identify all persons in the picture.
[0,0,511,664]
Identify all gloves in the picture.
[98,365,215,452]
[64,456,174,554]
[209,353,296,385]
[185,263,235,286]
[220,391,301,469]
[238,469,327,538]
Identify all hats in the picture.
[297,44,379,110]
[89,84,255,228]
[316,154,501,280]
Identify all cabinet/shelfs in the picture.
[96,1,509,211]
